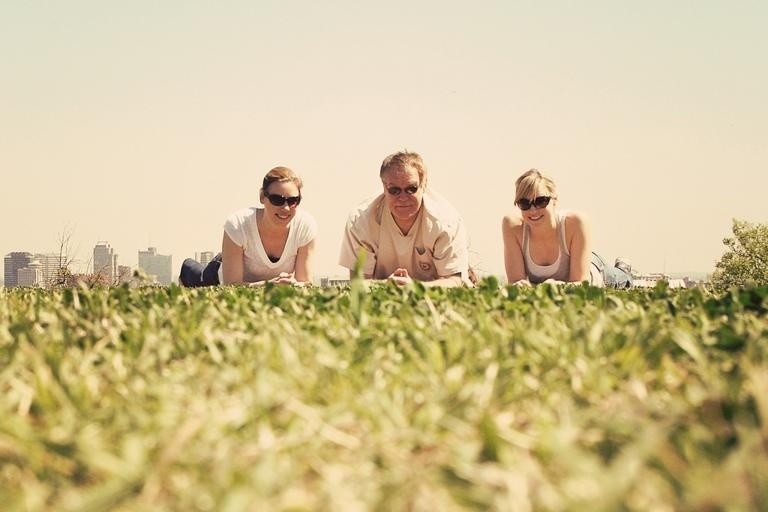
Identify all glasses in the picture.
[515,196,553,211]
[384,182,417,195]
[263,191,300,209]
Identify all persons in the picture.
[179,166,316,288]
[498,167,634,290]
[337,149,470,291]
